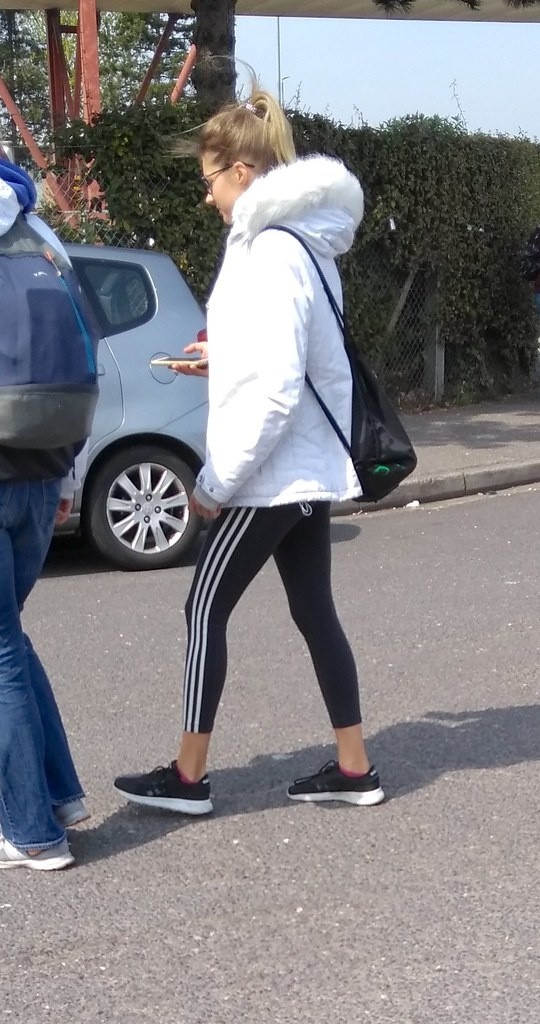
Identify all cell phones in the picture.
[151,357,208,368]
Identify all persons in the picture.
[0,144,100,871]
[111,92,386,816]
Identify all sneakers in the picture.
[0,828,75,870]
[113,760,213,814]
[288,761,385,805]
[54,799,90,827]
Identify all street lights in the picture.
[282,76,289,110]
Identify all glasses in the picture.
[200,160,255,187]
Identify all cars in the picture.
[51,242,209,571]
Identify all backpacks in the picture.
[260,225,417,502]
[0,215,102,452]
